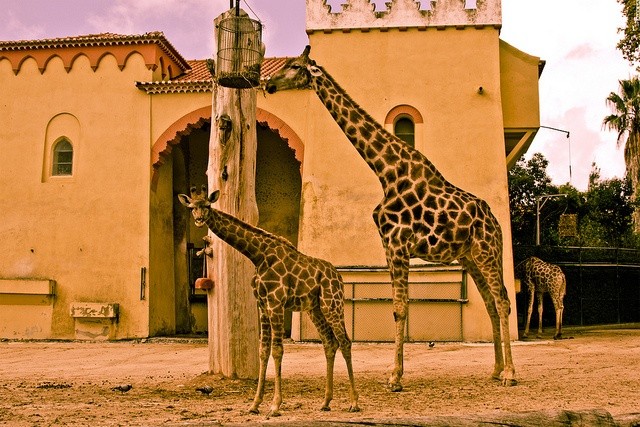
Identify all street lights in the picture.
[535,194,568,246]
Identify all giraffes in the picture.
[266,45,517,393]
[514,255,567,340]
[178,184,360,417]
